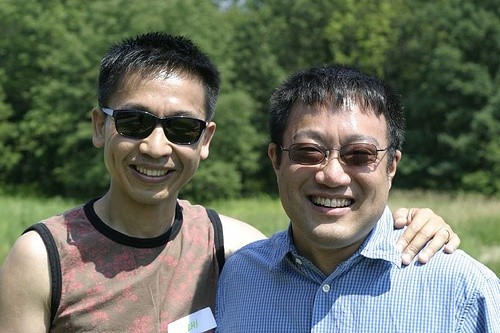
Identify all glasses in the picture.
[100,107,208,145]
[279,141,391,166]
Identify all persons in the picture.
[0,33,461,332]
[210,63,499,332]
